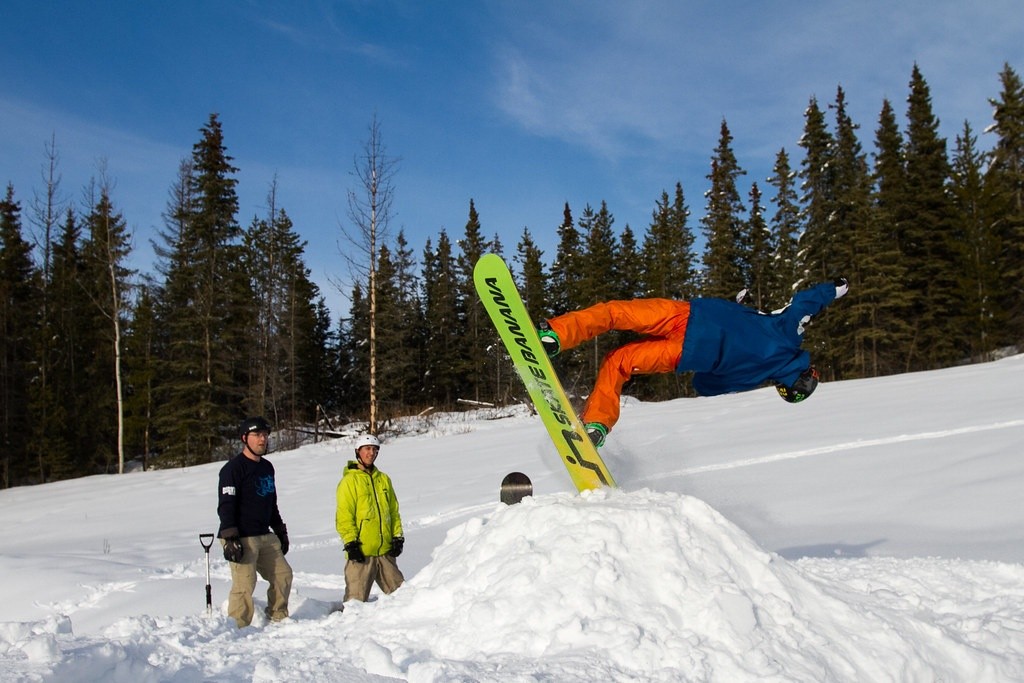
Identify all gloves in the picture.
[342,541,365,563]
[387,537,405,557]
[222,536,244,562]
[833,274,850,299]
[274,523,290,555]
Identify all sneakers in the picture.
[581,421,609,448]
[535,319,561,359]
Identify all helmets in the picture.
[354,434,380,450]
[775,366,820,404]
[238,416,272,439]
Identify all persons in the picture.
[335,435,407,605]
[537,275,849,448]
[219,417,294,627]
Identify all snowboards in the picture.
[472,251,620,495]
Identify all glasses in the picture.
[248,432,269,438]
